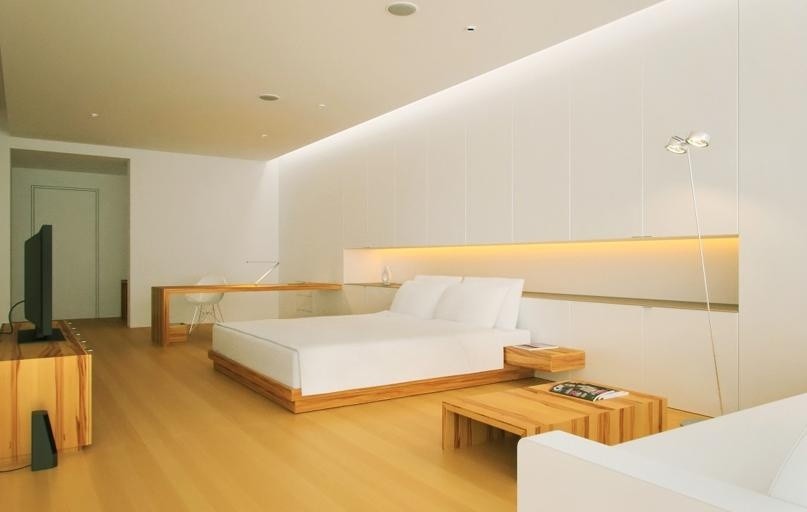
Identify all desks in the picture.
[151,284,343,348]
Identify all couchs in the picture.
[517,393,806,512]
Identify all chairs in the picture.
[185,274,230,335]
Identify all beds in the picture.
[210,312,532,413]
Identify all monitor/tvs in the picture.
[17,225,66,343]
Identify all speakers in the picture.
[32,410,57,471]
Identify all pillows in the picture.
[435,283,507,328]
[389,279,447,316]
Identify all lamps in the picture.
[666,131,729,414]
[239,259,281,285]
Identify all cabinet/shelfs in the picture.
[0,318,91,471]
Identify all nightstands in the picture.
[506,343,586,371]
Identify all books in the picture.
[515,343,560,351]
[549,382,630,402]
